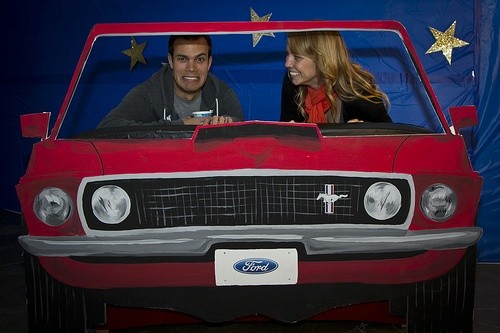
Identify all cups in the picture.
[193,110,214,118]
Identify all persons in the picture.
[97,35,244,128]
[280,29,392,125]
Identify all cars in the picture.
[15,20,486,330]
